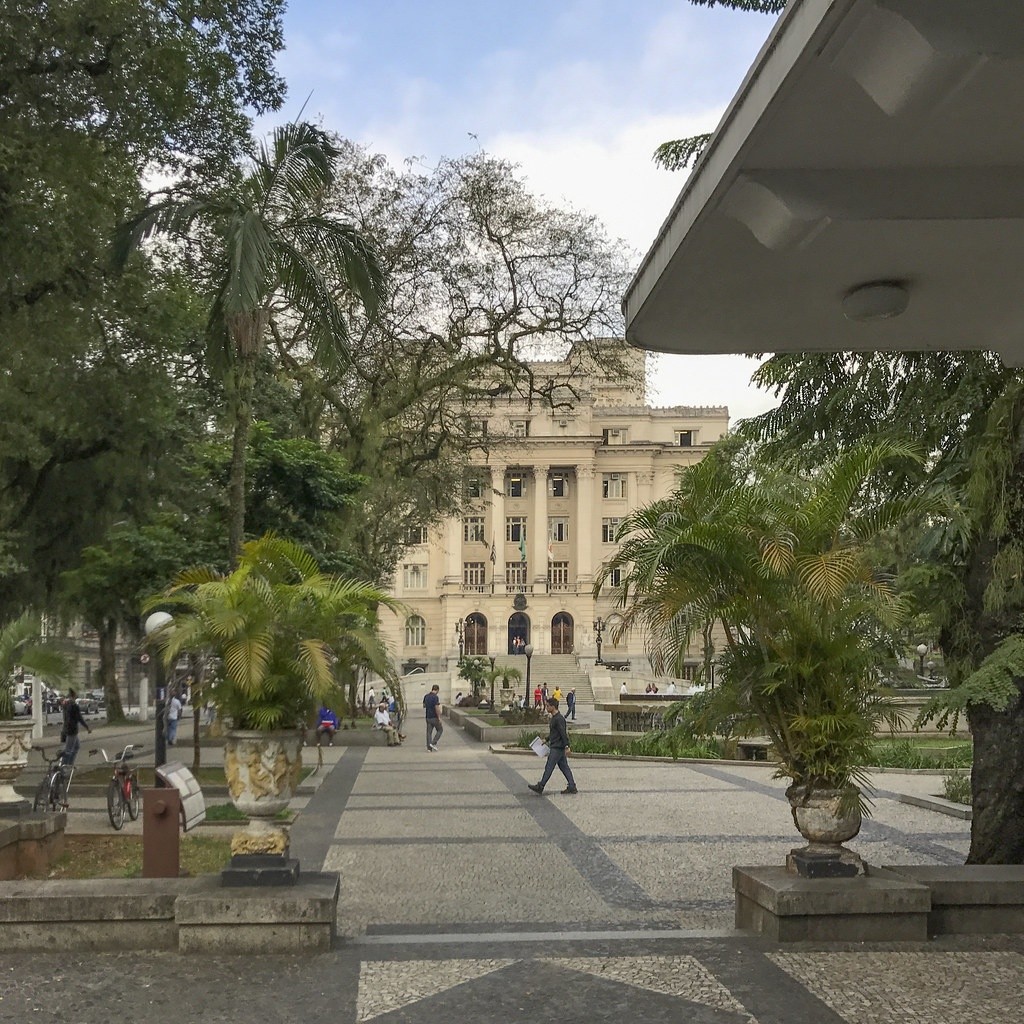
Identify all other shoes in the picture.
[430,744,438,751]
[428,750,432,752]
[528,784,542,795]
[561,787,578,794]
[329,742,332,746]
[316,742,321,747]
[388,734,406,747]
[572,718,577,720]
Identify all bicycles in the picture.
[32,745,71,828]
[89,742,147,831]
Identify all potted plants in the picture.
[457,655,521,713]
[131,530,420,856]
[590,421,972,881]
[0,605,82,805]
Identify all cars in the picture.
[10,690,107,715]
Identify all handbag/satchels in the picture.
[60,731,66,742]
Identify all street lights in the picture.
[485,651,500,715]
[522,644,534,710]
[593,616,606,665]
[916,644,927,676]
[473,659,481,705]
[455,616,467,668]
[146,611,177,790]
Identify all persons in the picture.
[528,698,578,794]
[60,687,92,769]
[315,705,338,747]
[454,682,577,720]
[513,635,526,654]
[621,681,676,695]
[423,685,443,752]
[367,685,406,746]
[163,693,181,744]
